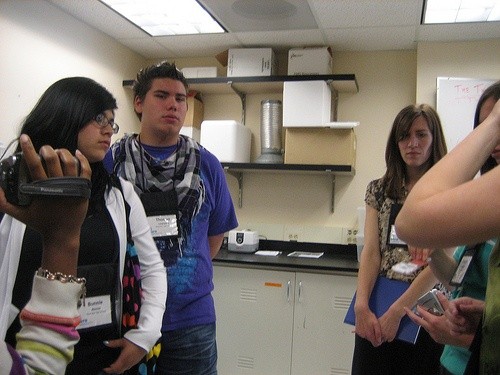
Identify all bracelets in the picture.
[37,268,87,284]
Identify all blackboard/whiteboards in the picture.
[436,76,500,180]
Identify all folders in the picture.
[344,276,421,344]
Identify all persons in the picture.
[351,105,450,375]
[394,78,500,375]
[0,134,92,375]
[102,60,237,375]
[0,78,169,375]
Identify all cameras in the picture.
[417,287,445,317]
[0,150,110,218]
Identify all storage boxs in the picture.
[215,47,335,78]
[283,81,356,170]
[178,91,251,164]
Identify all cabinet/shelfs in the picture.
[122,72,359,212]
[210,265,361,375]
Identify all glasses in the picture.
[91,113,119,134]
